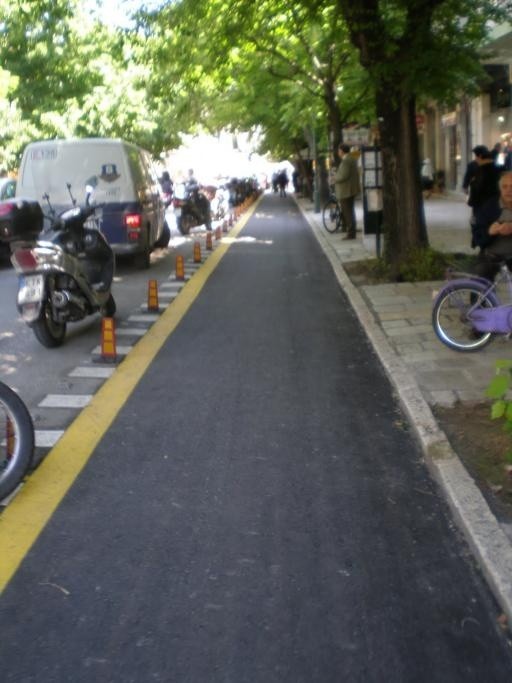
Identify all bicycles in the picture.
[432,250,512,351]
[321,190,348,233]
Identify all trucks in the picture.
[13,134,171,268]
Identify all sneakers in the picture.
[342,231,356,239]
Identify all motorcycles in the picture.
[0,380,36,501]
[157,174,260,234]
[0,182,117,347]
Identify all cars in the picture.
[0,176,17,264]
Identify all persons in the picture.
[461,143,511,339]
[329,144,360,239]
